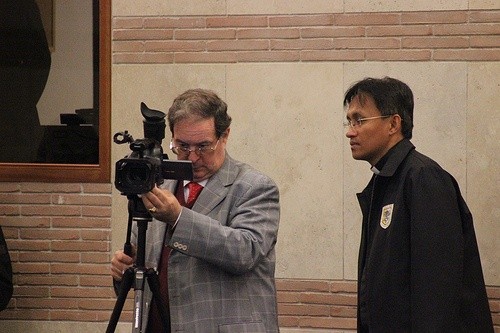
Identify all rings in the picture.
[149,206,156,213]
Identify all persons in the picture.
[342,76,494,333]
[0,0,51,163]
[110,88,281,333]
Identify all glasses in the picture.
[170,138,220,159]
[343,115,403,128]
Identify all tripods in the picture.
[105,195,171,333]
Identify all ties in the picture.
[187,183,202,205]
